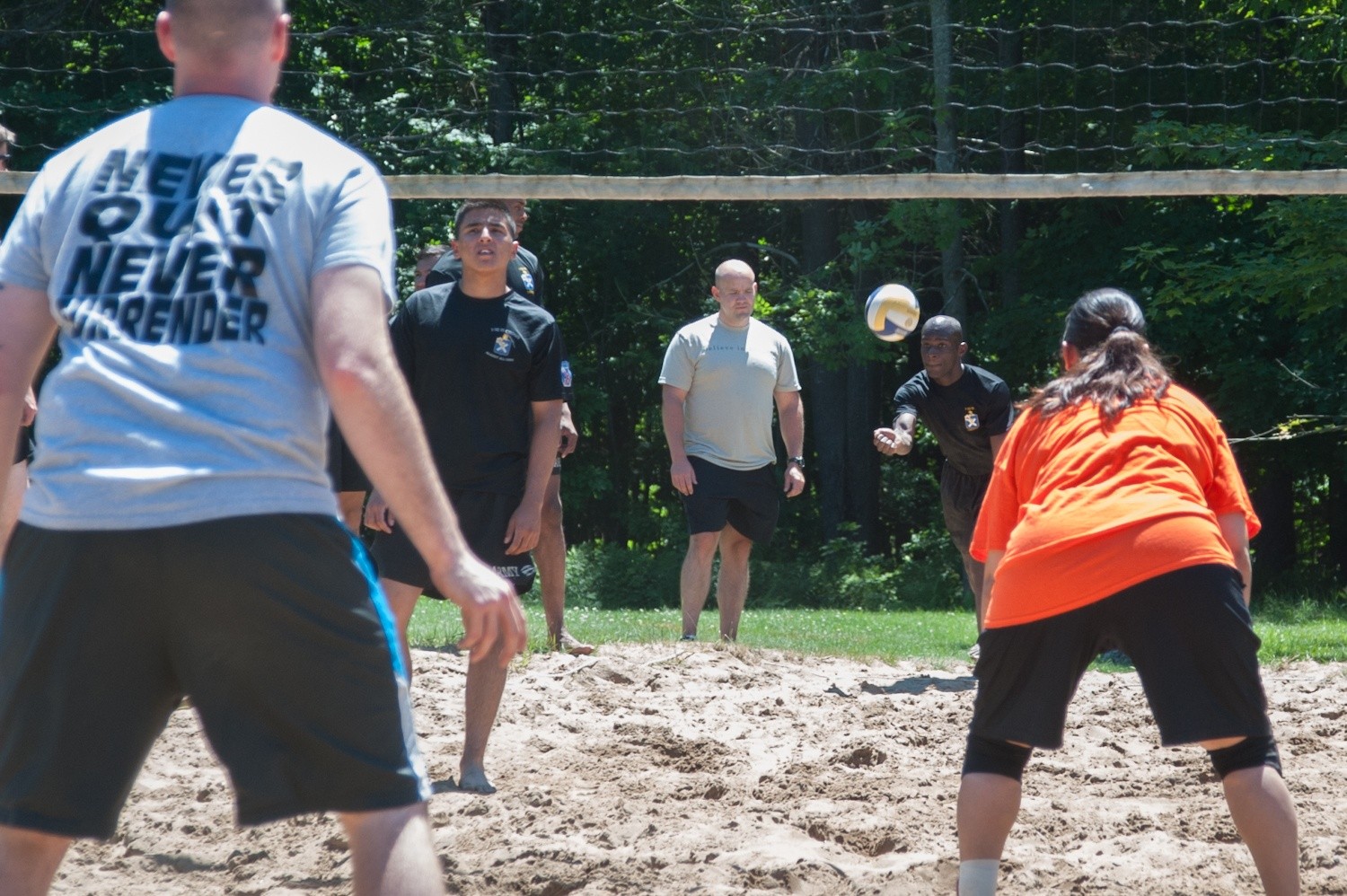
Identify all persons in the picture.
[324,403,367,539]
[412,242,451,297]
[873,314,1018,663]
[659,258,806,647]
[355,203,567,798]
[957,288,1304,896]
[429,182,598,658]
[1,0,535,895]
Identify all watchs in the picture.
[786,453,804,469]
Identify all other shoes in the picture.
[969,636,980,659]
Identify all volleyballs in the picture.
[864,282,922,343]
